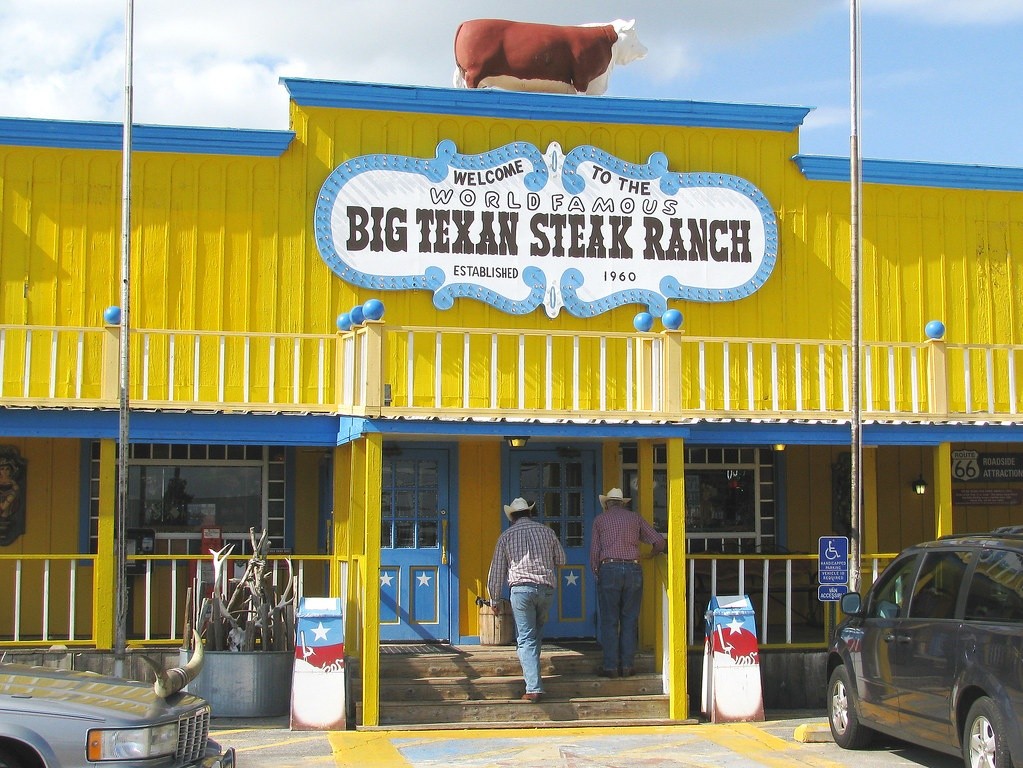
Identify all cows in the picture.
[453,18,649,99]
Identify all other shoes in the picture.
[522,693,542,703]
[621,668,636,677]
[598,666,618,678]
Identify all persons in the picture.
[487,497,566,703]
[590,488,666,679]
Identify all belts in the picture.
[513,582,553,590]
[602,558,640,564]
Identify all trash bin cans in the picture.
[287,596,348,731]
[700,594,767,725]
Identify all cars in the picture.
[0,632,242,767]
[824,525,1022,768]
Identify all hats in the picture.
[599,488,631,510]
[504,497,535,521]
